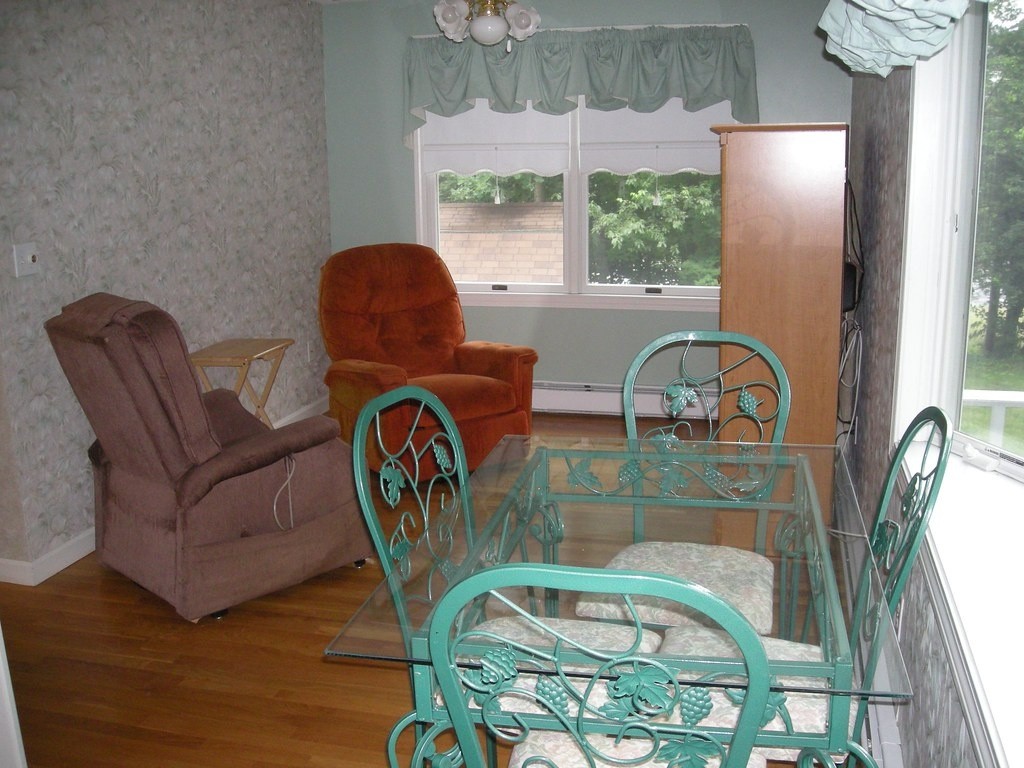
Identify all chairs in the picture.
[631,407,953,768]
[576,330,792,637]
[429,562,772,768]
[352,386,661,768]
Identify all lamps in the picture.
[433,0,542,52]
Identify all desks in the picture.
[190,339,296,430]
[324,434,913,768]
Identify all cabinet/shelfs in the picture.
[710,122,851,558]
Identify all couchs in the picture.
[43,292,374,623]
[319,243,539,486]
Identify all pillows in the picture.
[202,387,269,448]
